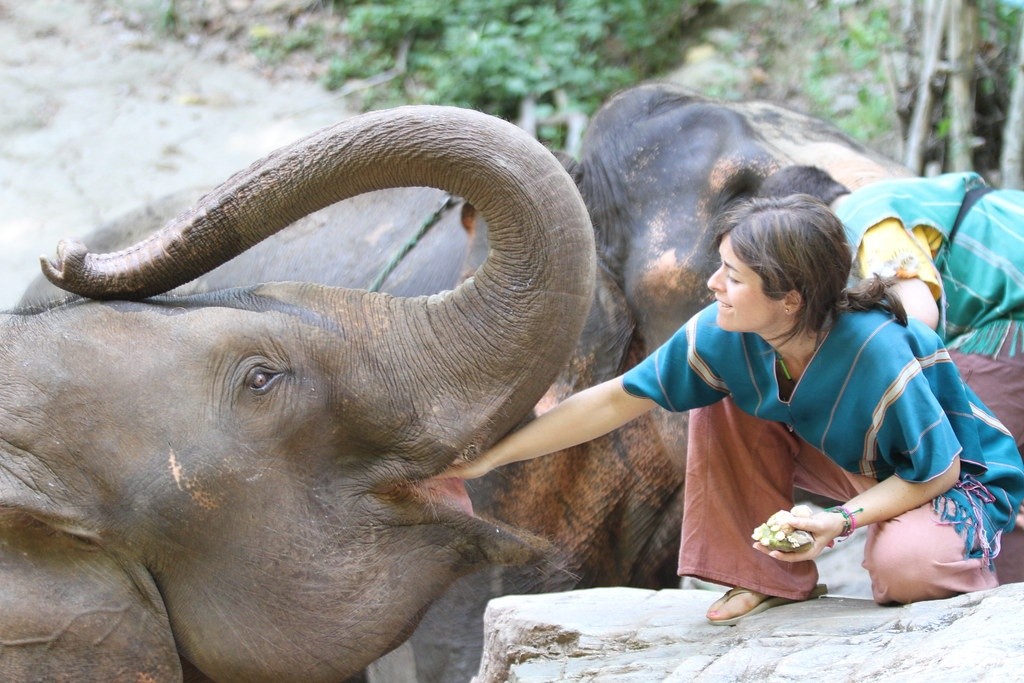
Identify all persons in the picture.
[757,165,1024,587]
[439,194,1024,628]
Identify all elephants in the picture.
[0,76,1024,683]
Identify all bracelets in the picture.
[824,506,863,542]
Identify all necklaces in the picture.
[774,331,821,388]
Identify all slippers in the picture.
[707,583,828,625]
